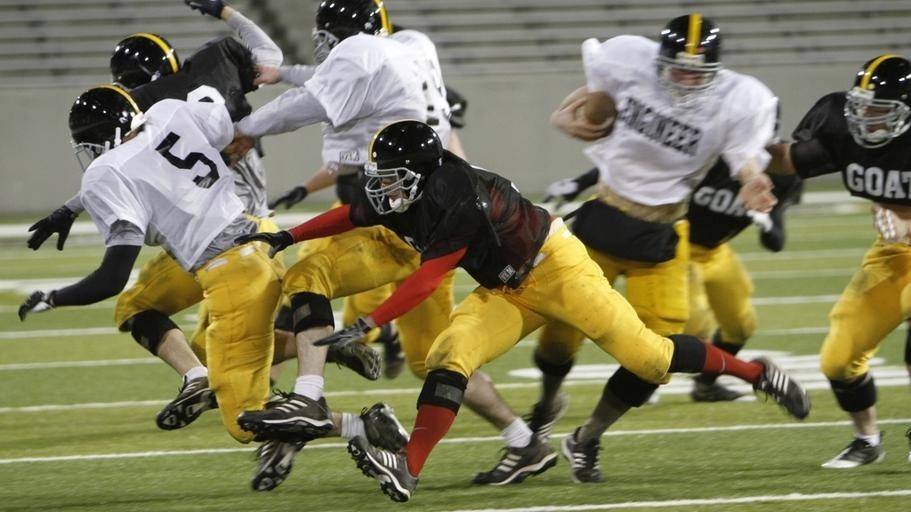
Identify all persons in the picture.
[15,1,911,507]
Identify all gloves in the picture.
[27,206,78,250]
[184,1,225,19]
[315,321,370,351]
[233,231,292,259]
[747,209,773,233]
[267,187,306,211]
[17,289,56,323]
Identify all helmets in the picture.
[68,84,144,170]
[312,1,391,61]
[111,33,180,89]
[364,119,442,214]
[651,15,721,115]
[843,56,911,149]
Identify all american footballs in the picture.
[574,90,615,138]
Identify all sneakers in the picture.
[249,440,306,491]
[564,428,603,484]
[383,324,404,381]
[237,394,332,439]
[528,396,568,433]
[823,432,886,470]
[689,381,754,404]
[329,340,381,381]
[358,402,410,454]
[350,436,420,501]
[155,378,219,430]
[750,357,810,419]
[473,434,558,486]
